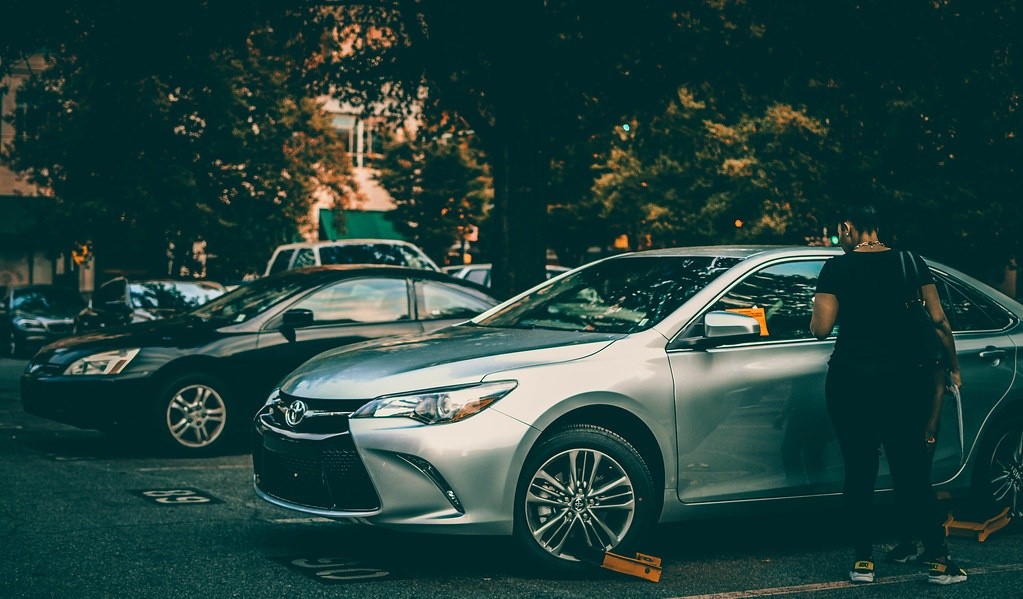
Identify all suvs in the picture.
[256,235,607,309]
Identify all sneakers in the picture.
[928,560,967,585]
[849,555,875,582]
[886,542,917,563]
[917,543,951,565]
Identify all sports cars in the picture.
[252,242,1023,581]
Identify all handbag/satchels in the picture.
[899,250,944,373]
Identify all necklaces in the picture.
[851,241,886,250]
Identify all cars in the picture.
[0,283,89,357]
[19,263,659,461]
[86,275,232,328]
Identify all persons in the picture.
[810,203,968,584]
[822,220,841,247]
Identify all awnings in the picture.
[319,209,402,240]
[0,195,72,247]
